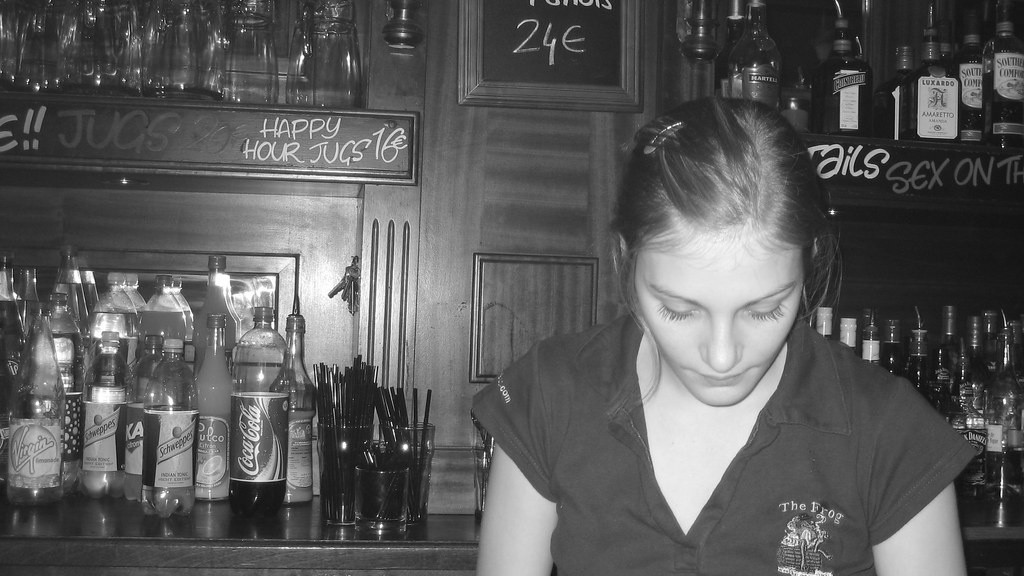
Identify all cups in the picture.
[0,1,365,113]
[316,418,436,535]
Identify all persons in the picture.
[469,98,973,576]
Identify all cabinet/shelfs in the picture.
[0,0,1024,575]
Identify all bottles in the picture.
[802,299,1024,514]
[716,0,1024,150]
[0,243,312,517]
